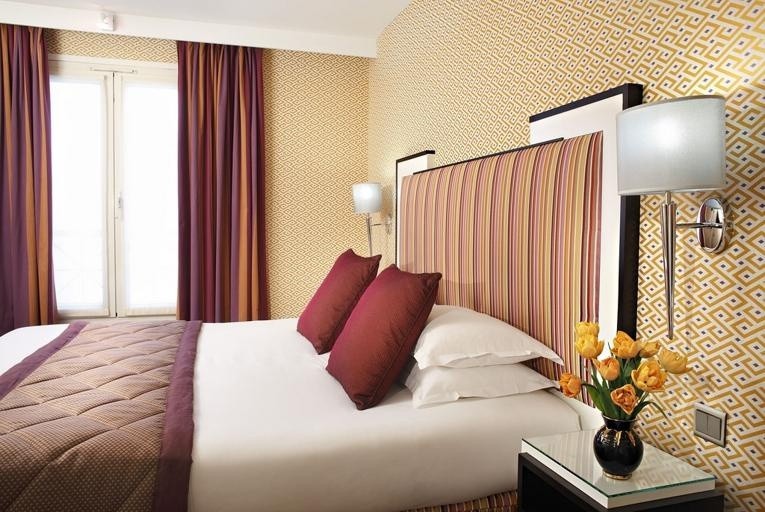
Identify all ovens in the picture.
[0,84,641,512]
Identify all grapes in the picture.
[516,429,724,512]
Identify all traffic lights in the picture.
[296,249,381,354]
[325,264,442,411]
[406,362,561,412]
[410,303,565,373]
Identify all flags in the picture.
[593,413,644,480]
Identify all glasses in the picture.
[616,95,730,338]
[352,183,392,259]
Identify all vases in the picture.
[559,320,694,431]
